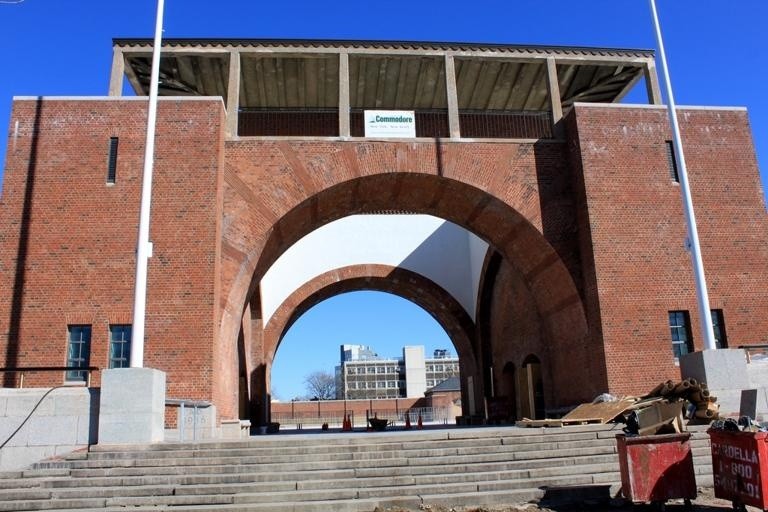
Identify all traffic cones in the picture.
[415,415,425,430]
[403,417,413,431]
[341,419,353,431]
[322,422,329,431]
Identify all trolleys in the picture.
[368,418,393,431]
